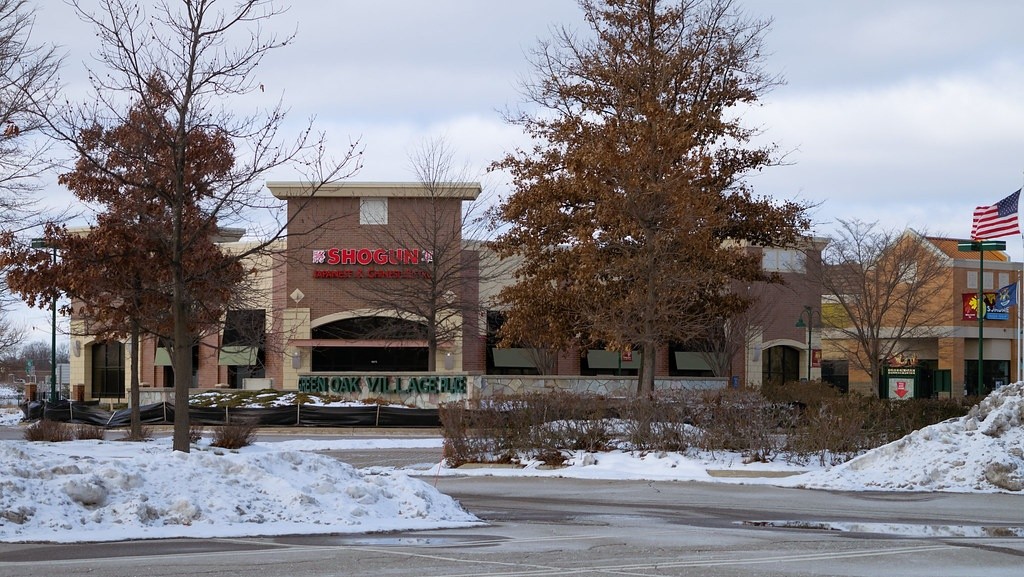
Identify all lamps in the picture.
[71,341,80,357]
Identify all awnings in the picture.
[674,352,732,372]
[493,348,540,367]
[218,346,265,368]
[587,349,642,370]
[153,347,174,366]
[971,188,1021,241]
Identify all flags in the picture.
[996,281,1020,309]
